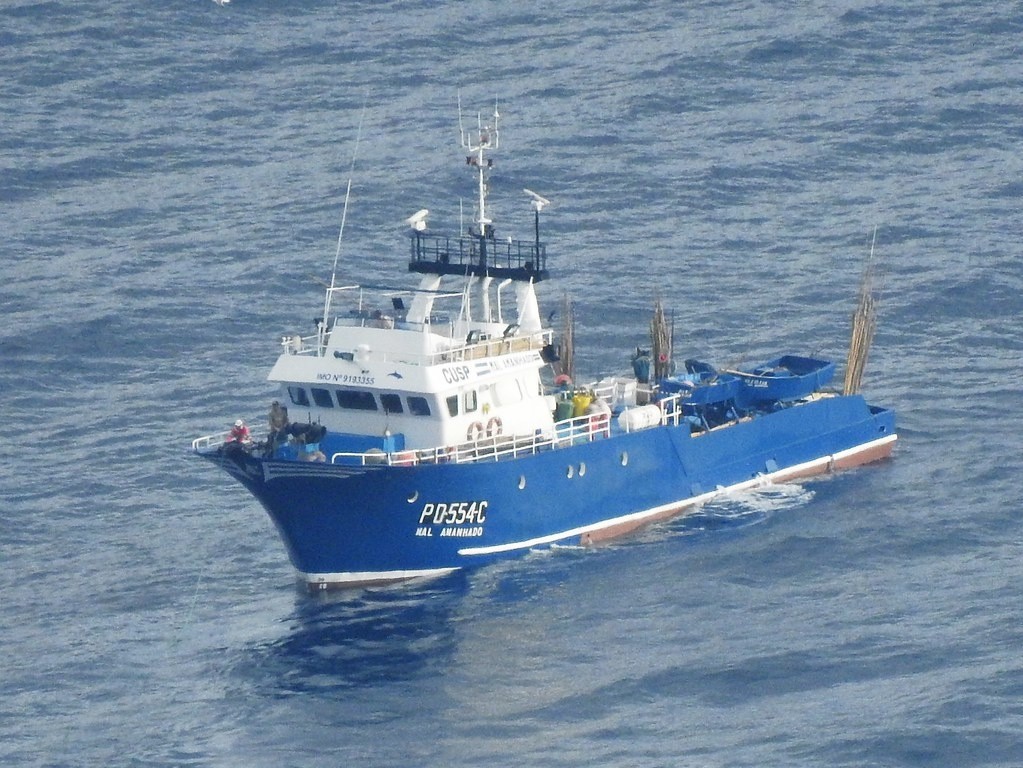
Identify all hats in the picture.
[235,420,242,426]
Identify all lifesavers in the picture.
[486,417,502,437]
[467,422,482,441]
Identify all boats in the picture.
[188,82,899,589]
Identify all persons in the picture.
[225,419,252,443]
[268,401,286,437]
